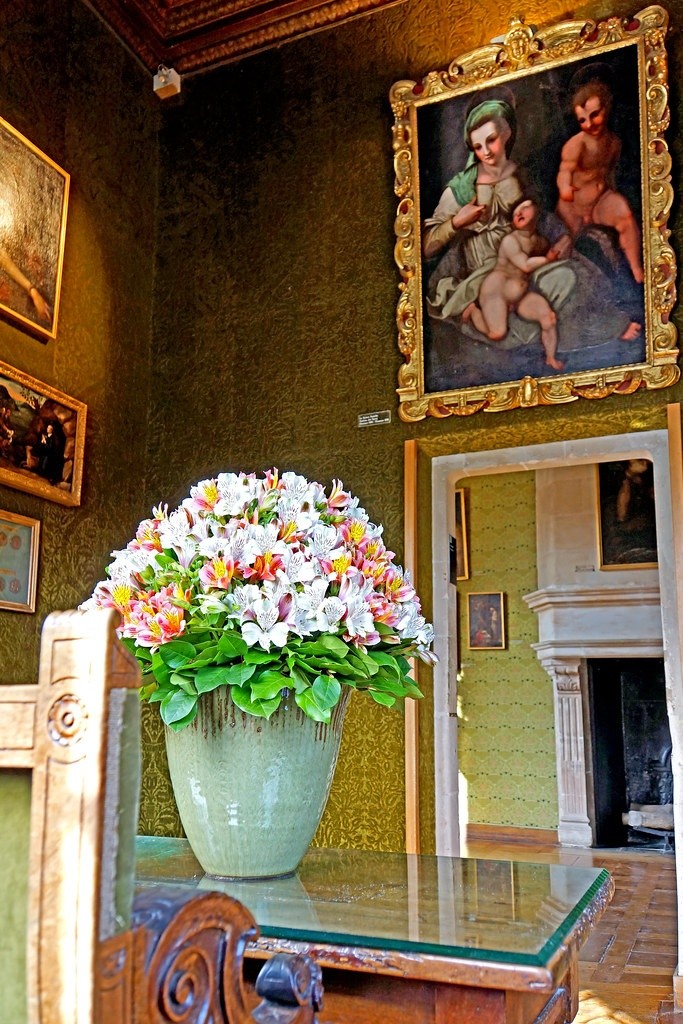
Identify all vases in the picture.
[160,680,354,880]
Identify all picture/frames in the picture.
[387,5,682,424]
[592,458,659,571]
[0,116,71,339]
[0,360,87,507]
[455,488,470,582]
[0,508,41,614]
[466,592,507,650]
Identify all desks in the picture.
[137,835,617,1023]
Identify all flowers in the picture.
[79,465,442,729]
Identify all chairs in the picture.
[0,606,324,1024]
